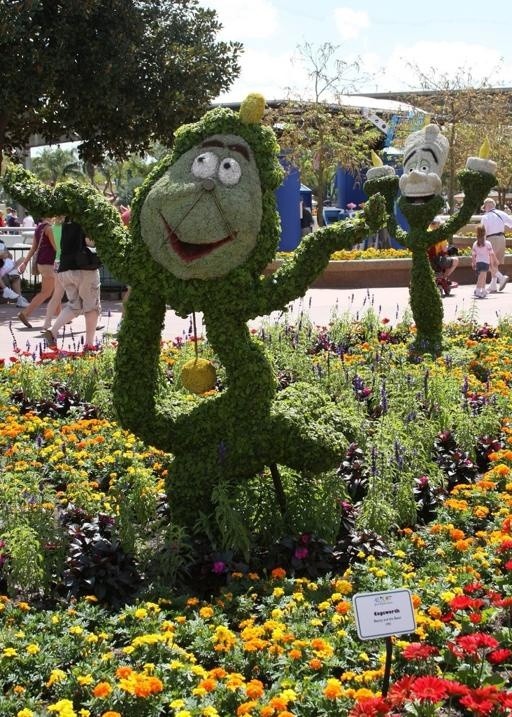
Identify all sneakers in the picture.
[474,276,508,298]
[16,312,32,328]
[2,287,31,307]
[44,330,57,351]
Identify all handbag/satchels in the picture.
[32,252,40,275]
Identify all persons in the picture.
[472,198,512,298]
[428,221,459,285]
[0,208,102,351]
[301,204,313,236]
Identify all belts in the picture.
[487,232,505,237]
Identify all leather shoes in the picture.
[96,324,105,330]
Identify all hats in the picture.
[481,198,496,210]
